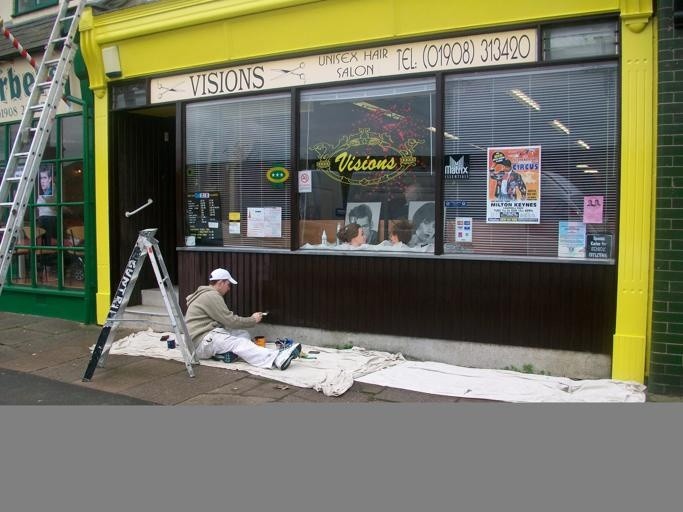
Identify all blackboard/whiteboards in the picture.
[188,193,223,247]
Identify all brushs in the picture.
[265,313,269,318]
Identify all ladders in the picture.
[82,229,201,379]
[1,1,88,294]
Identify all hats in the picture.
[208,268,238,285]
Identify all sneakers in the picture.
[273,342,302,371]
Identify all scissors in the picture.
[277,337,289,351]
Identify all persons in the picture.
[389,216,417,246]
[490,159,527,201]
[587,200,593,206]
[36,171,74,266]
[348,204,377,244]
[40,167,52,195]
[594,199,601,206]
[183,267,303,371]
[337,223,366,246]
[413,201,435,243]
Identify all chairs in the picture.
[16,223,83,288]
